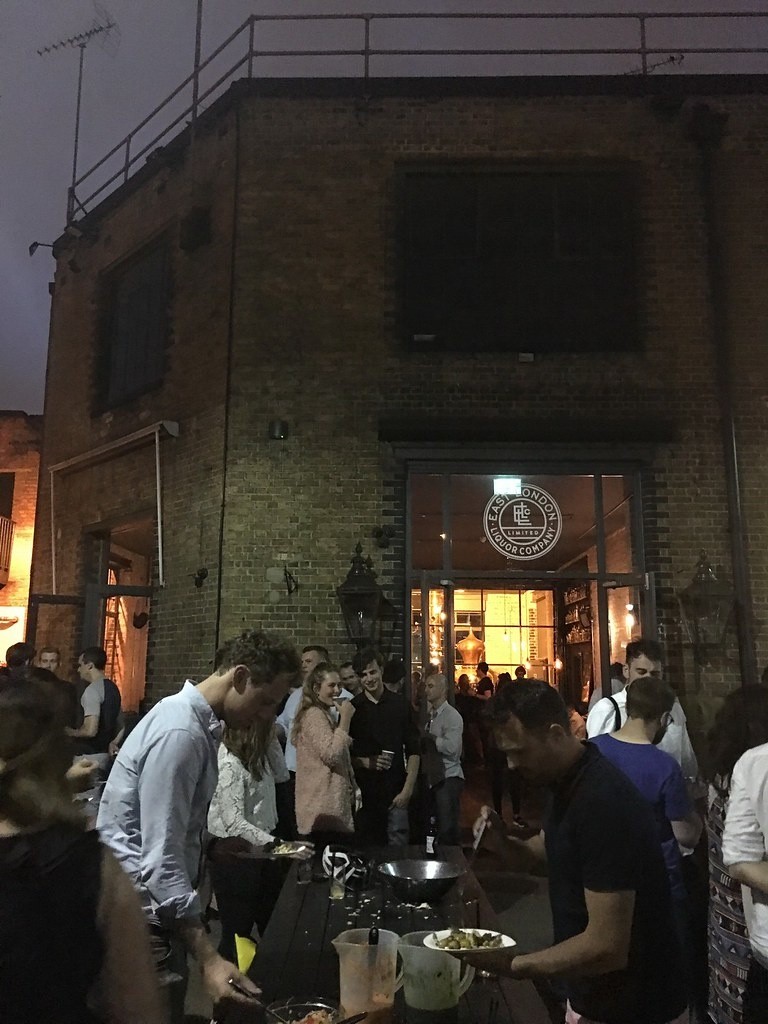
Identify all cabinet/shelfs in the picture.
[559,578,591,645]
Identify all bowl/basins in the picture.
[266,996,345,1024]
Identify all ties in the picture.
[425,718,432,734]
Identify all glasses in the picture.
[666,713,674,727]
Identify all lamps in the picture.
[29,241,54,256]
[652,74,698,113]
[337,540,385,654]
[677,546,739,669]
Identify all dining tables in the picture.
[222,843,552,1024]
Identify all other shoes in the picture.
[512,815,529,827]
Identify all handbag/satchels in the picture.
[86,941,187,1022]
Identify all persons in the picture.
[443,679,697,1024]
[693,666,768,1024]
[411,658,588,832]
[0,664,169,1024]
[581,677,702,983]
[2,640,126,804]
[204,645,468,964]
[97,629,304,1023]
[585,638,701,778]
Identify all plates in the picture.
[424,927,517,951]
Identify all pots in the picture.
[377,858,466,902]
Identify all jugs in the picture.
[394,931,475,1010]
[331,928,404,1024]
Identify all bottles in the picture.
[426,816,440,861]
